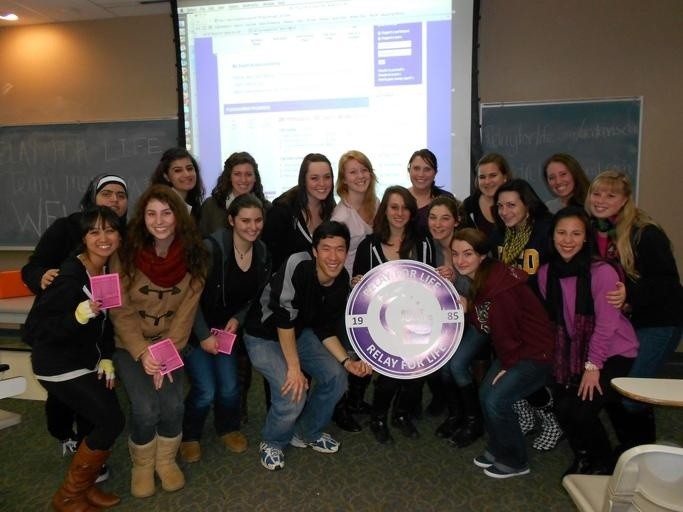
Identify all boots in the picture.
[156,432,183,491]
[512,398,537,436]
[532,389,563,451]
[54,439,121,511]
[127,433,156,498]
[334,374,482,446]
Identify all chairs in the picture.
[561,444,683,512]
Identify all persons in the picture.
[451,229,556,474]
[154,148,682,445]
[178,194,272,461]
[18,174,144,512]
[536,207,639,474]
[108,183,210,497]
[244,221,372,466]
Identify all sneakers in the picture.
[289,433,339,454]
[258,442,285,471]
[222,432,247,453]
[473,455,492,469]
[177,442,200,463]
[484,465,529,478]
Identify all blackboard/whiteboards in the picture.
[0,119,178,251]
[479,97,644,211]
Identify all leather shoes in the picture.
[568,454,590,474]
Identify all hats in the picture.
[97,174,127,196]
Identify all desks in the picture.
[611,376,682,406]
